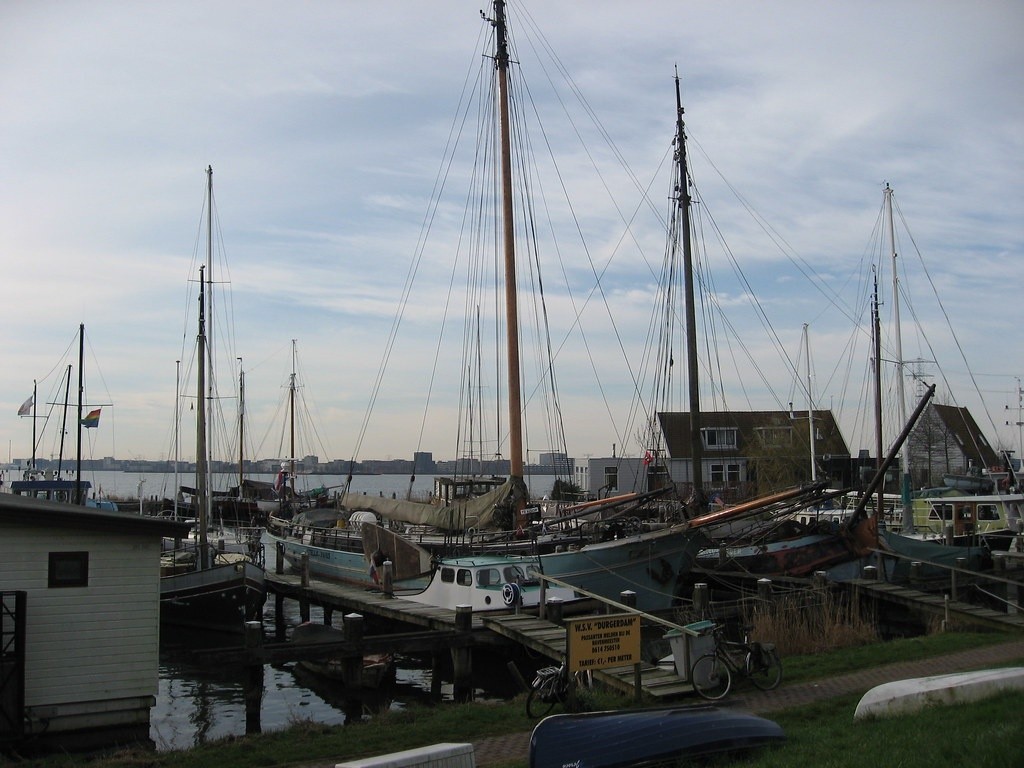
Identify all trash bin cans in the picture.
[663,619,720,690]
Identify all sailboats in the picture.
[162,165,255,553]
[266,0,861,632]
[160,265,266,633]
[10,323,118,511]
[169,339,345,520]
[528,61,1024,580]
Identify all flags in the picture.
[18,394,33,415]
[642,450,654,465]
[81,409,101,428]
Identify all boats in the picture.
[343,552,593,625]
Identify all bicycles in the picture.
[526,662,593,720]
[689,624,782,701]
